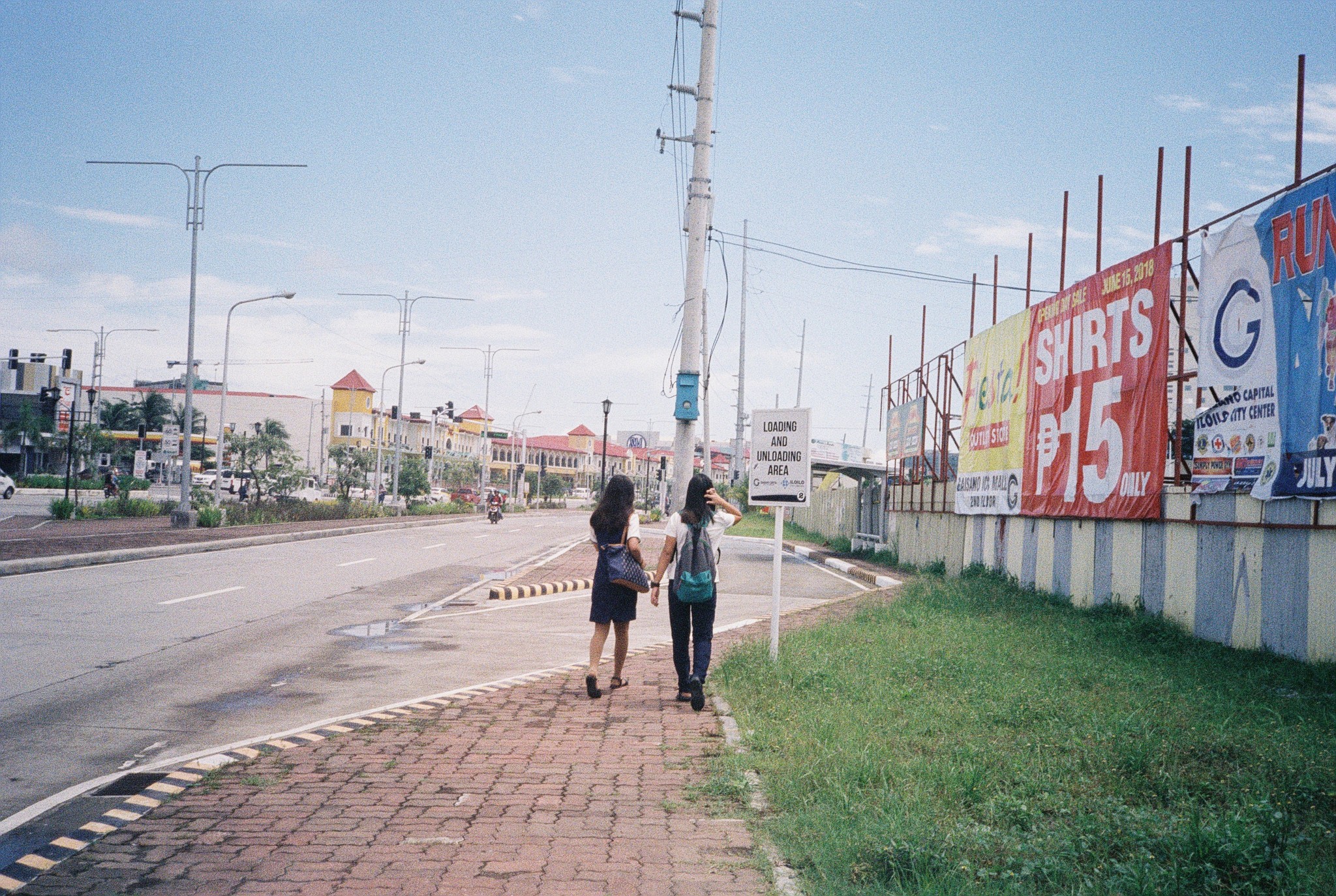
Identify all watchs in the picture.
[650,581,659,588]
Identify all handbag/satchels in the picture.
[601,512,650,594]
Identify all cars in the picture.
[0,468,15,500]
[409,485,503,507]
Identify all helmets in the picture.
[494,490,500,493]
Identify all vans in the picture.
[572,487,590,499]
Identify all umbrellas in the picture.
[499,489,509,494]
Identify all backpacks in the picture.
[672,509,716,603]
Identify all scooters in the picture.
[486,500,501,524]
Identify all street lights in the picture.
[338,291,476,512]
[46,155,322,521]
[508,410,542,507]
[84,387,97,469]
[214,290,297,507]
[373,360,427,502]
[644,451,651,515]
[600,399,613,501]
[439,344,540,505]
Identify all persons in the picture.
[664,496,671,517]
[243,478,253,498]
[650,474,742,711]
[106,470,119,495]
[502,494,507,504]
[483,489,489,515]
[487,490,504,520]
[586,475,646,699]
[377,483,387,504]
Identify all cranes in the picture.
[167,358,314,379]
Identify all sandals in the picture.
[609,677,628,688]
[585,675,602,698]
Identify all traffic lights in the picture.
[517,464,523,473]
[137,423,145,438]
[427,446,433,459]
[391,405,398,419]
[540,466,547,477]
[53,386,62,402]
[145,450,152,460]
[437,406,443,413]
[445,401,454,419]
[40,387,49,402]
[655,469,662,481]
[660,456,666,469]
[424,446,428,459]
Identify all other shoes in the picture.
[488,514,492,519]
[690,677,705,711]
[501,515,504,519]
[675,691,692,701]
